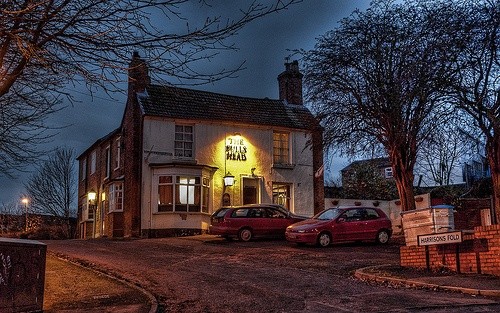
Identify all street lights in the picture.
[22,197,29,231]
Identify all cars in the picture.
[285,204,393,249]
[208,202,313,242]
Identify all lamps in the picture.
[223,172,235,186]
[234,132,242,138]
